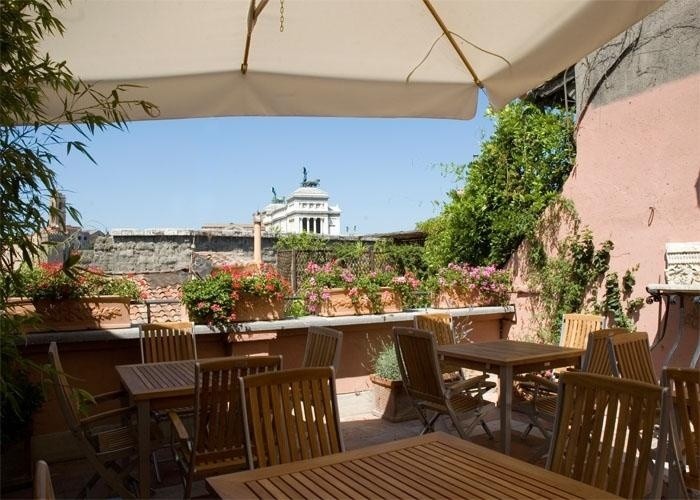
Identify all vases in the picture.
[306,284,406,316]
[187,296,286,321]
[4,294,132,334]
[434,283,505,307]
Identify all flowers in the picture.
[6,257,148,294]
[301,260,419,311]
[427,258,514,292]
[177,262,290,319]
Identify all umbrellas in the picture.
[0,1,670,125]
